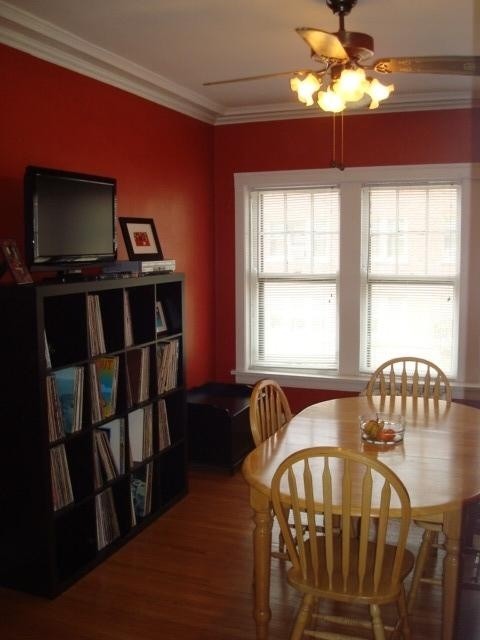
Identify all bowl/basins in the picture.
[360,414,405,446]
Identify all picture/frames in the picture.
[155,301,168,333]
[1,239,34,285]
[118,216,164,259]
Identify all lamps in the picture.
[288,59,394,113]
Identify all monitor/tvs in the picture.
[23,164,118,286]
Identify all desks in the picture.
[185,384,256,476]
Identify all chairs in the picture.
[249,379,365,559]
[272,445,415,639]
[367,356,452,402]
[405,506,480,615]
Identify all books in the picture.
[131,462,153,526]
[95,492,116,551]
[47,366,85,440]
[50,443,75,510]
[127,404,153,468]
[92,417,125,488]
[155,339,178,394]
[131,347,151,404]
[89,358,119,424]
[157,398,172,451]
[125,293,135,345]
[87,295,107,356]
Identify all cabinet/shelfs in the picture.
[1,272,188,604]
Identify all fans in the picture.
[203,1,480,87]
